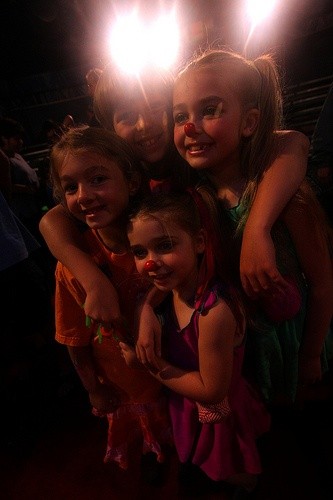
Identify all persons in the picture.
[0,120,43,378]
[48,122,189,485]
[39,58,311,328]
[118,185,277,499]
[173,50,333,500]
